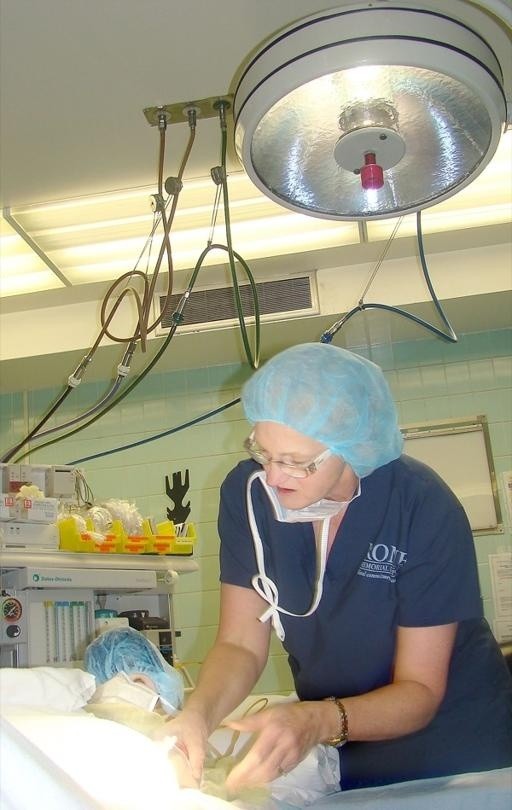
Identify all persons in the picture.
[94,664,200,807]
[163,340,512,792]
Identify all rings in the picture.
[278,765,288,777]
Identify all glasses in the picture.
[243,426,333,479]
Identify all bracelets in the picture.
[323,696,349,749]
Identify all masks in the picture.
[257,469,363,524]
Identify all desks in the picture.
[1,556,200,692]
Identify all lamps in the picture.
[233,0,508,222]
